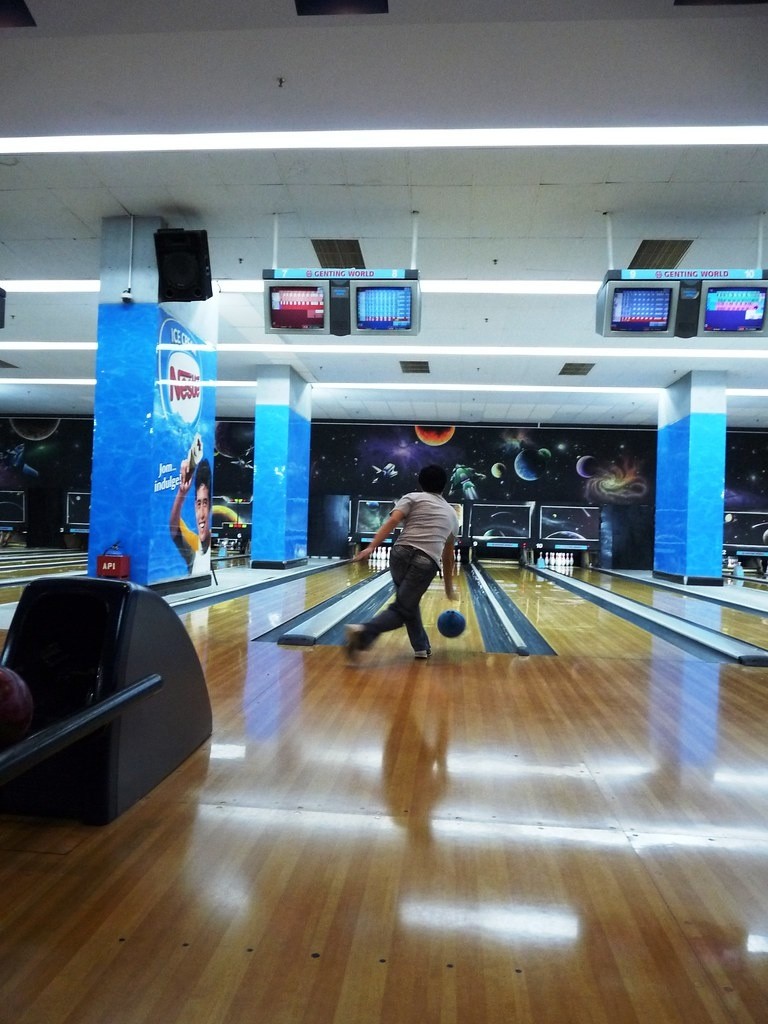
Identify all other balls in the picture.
[0,666,35,746]
[437,609,466,637]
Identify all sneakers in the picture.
[343,624,366,669]
[414,649,432,658]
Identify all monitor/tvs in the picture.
[595,281,680,338]
[264,279,329,336]
[350,280,421,336]
[696,279,768,338]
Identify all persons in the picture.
[344,464,459,659]
[169,460,212,576]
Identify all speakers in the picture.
[154,226,214,302]
